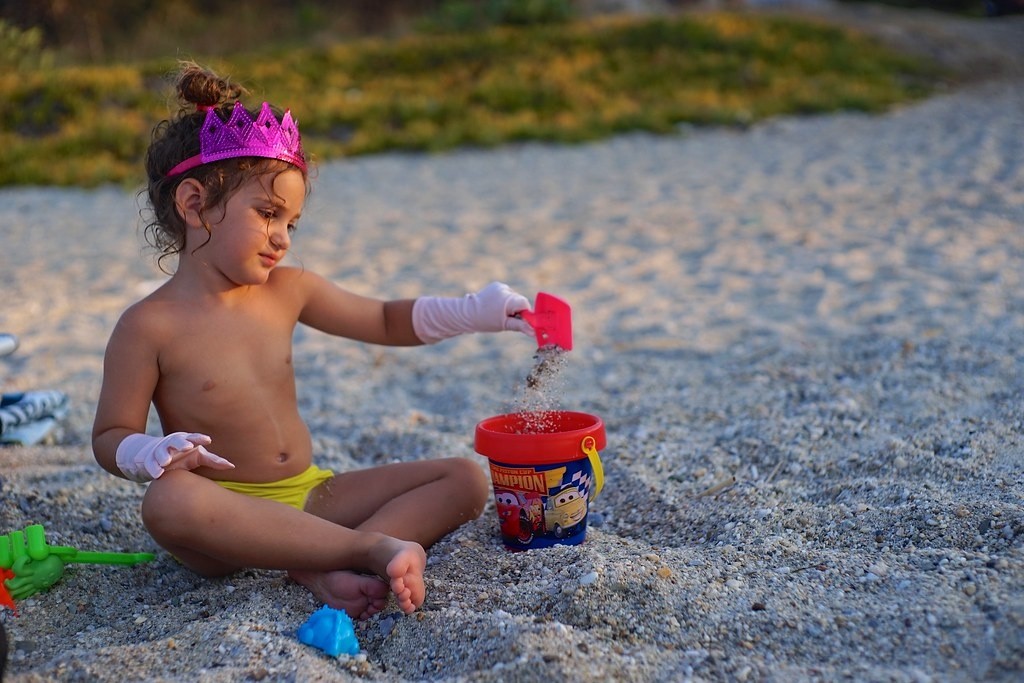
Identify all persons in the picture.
[91,58,536,621]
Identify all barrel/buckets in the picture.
[475,412,605,552]
